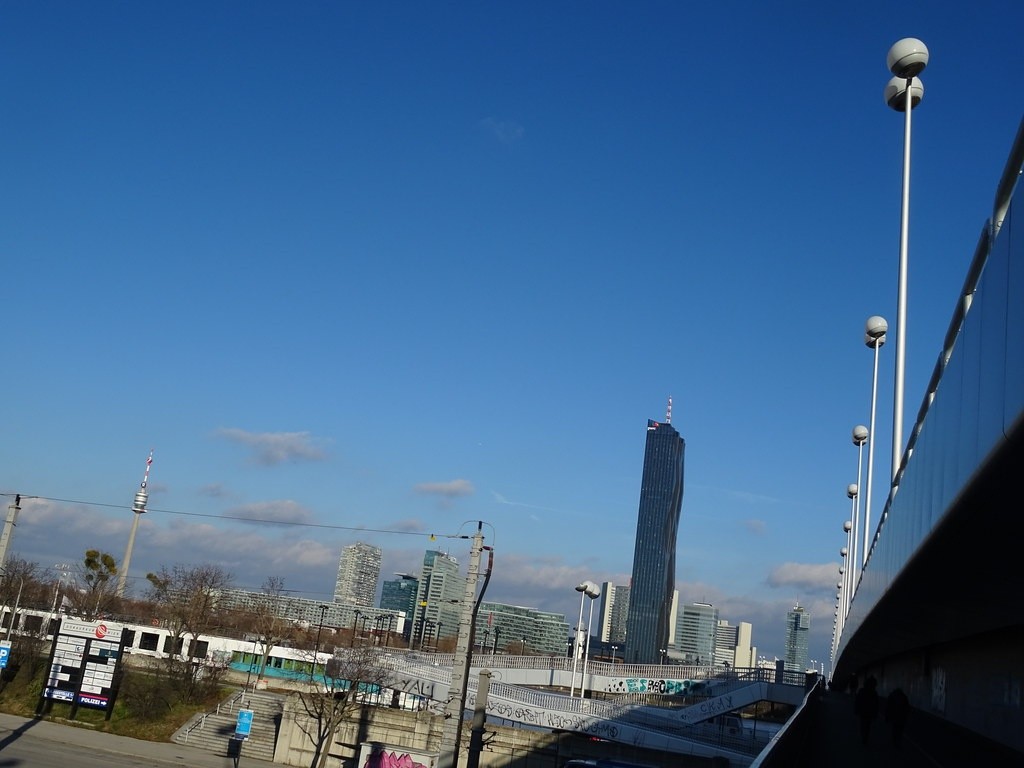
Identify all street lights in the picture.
[612,646,618,662]
[861,315,889,572]
[759,655,765,668]
[52,573,67,608]
[566,643,571,657]
[829,426,869,667]
[810,659,817,671]
[659,649,666,665]
[570,580,600,711]
[884,37,930,484]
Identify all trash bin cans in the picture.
[226,737,243,757]
